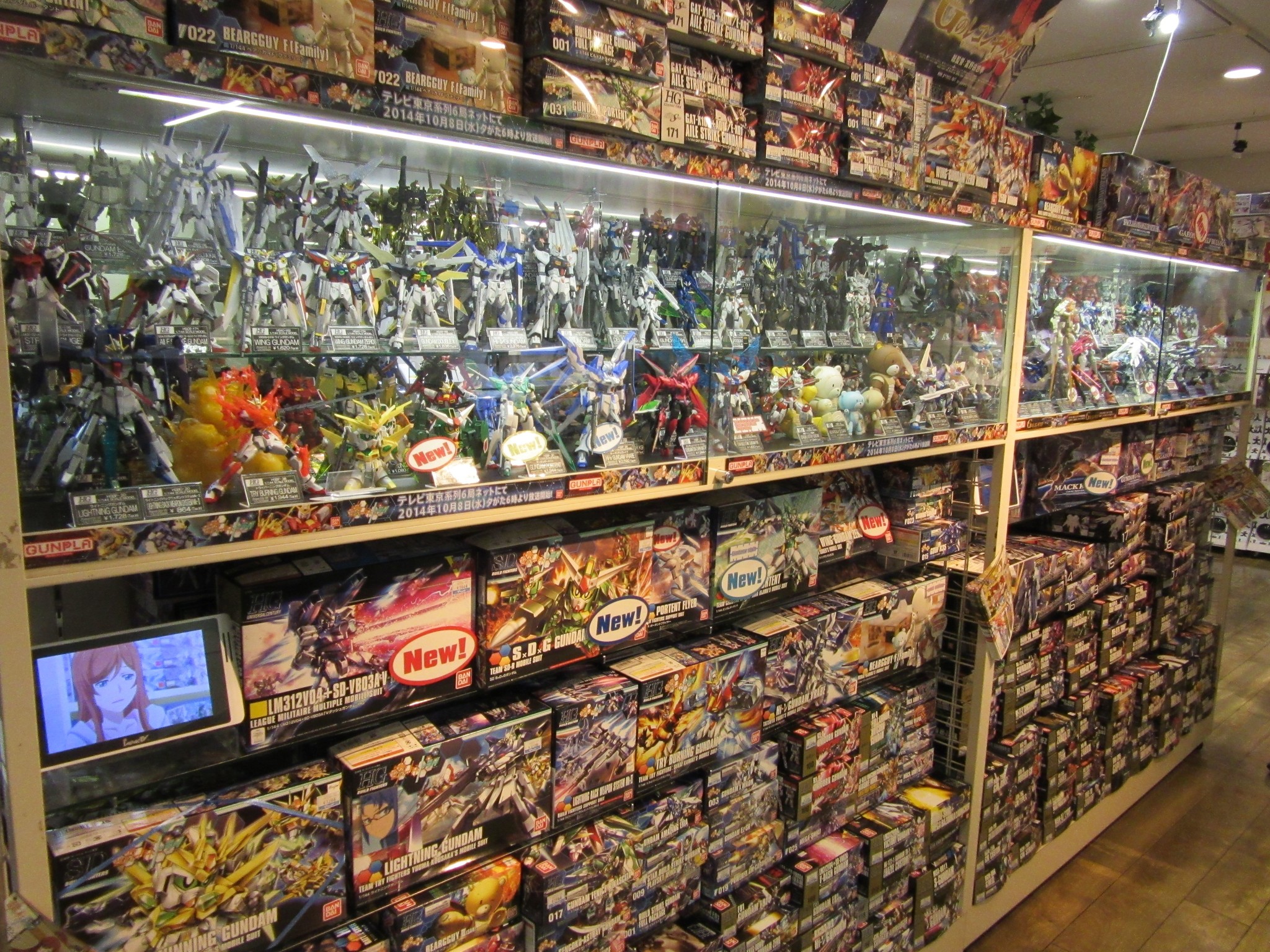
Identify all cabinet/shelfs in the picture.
[0,39,1270,952]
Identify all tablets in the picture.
[32,612,246,772]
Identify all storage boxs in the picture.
[0,0,1270,272]
[48,405,1232,952]
